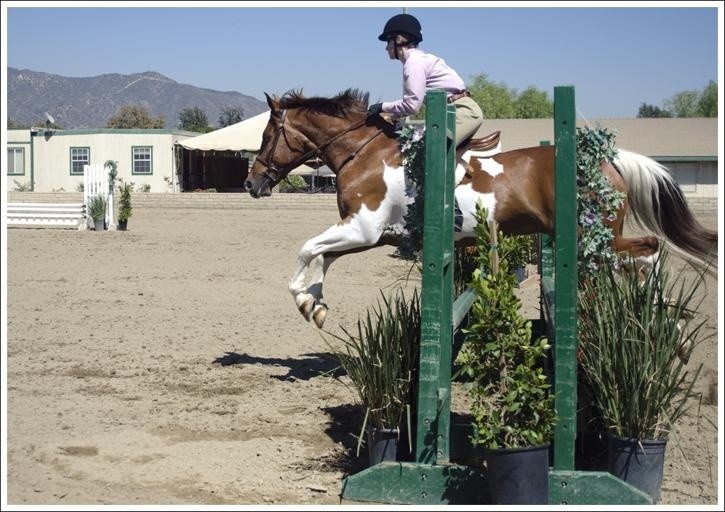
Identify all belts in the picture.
[448,90,470,104]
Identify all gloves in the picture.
[367,103,383,118]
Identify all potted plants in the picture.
[577,230,721,505]
[312,282,417,471]
[451,200,557,505]
[453,239,523,296]
[85,180,133,230]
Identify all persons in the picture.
[369,14,484,149]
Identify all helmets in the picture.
[378,14,423,42]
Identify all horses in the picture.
[243,87,718,366]
